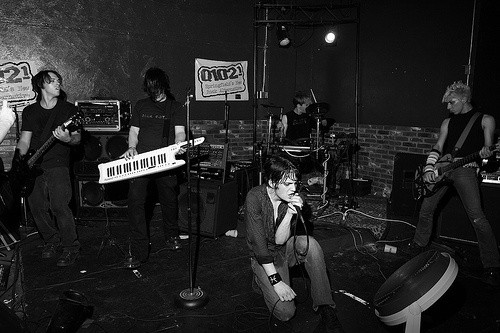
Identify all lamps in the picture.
[276,24,290,47]
[324,26,337,43]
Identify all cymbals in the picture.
[305,102,331,117]
[260,102,286,109]
[265,113,280,120]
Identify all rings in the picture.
[482,155,484,157]
[57,134,59,136]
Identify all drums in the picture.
[257,132,357,192]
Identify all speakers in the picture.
[73,177,128,221]
[75,133,130,177]
[434,182,500,281]
[390,153,428,215]
[177,180,238,240]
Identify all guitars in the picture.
[4,110,84,198]
[414,141,500,199]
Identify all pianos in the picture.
[97,136,207,185]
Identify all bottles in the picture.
[346,169,349,179]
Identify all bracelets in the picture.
[268,272,282,286]
[129,146,136,149]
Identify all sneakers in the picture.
[252,273,262,295]
[318,304,345,333]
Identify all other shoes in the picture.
[169,235,183,250]
[407,240,422,249]
[484,266,500,286]
[56,248,80,267]
[41,243,61,259]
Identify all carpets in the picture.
[239,193,392,241]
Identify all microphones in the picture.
[291,194,305,223]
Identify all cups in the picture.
[383,244,397,254]
[225,230,238,237]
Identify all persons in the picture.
[398,80,500,286]
[246,156,344,333]
[12,70,84,266]
[0,100,17,201]
[280,91,314,175]
[118,68,187,271]
